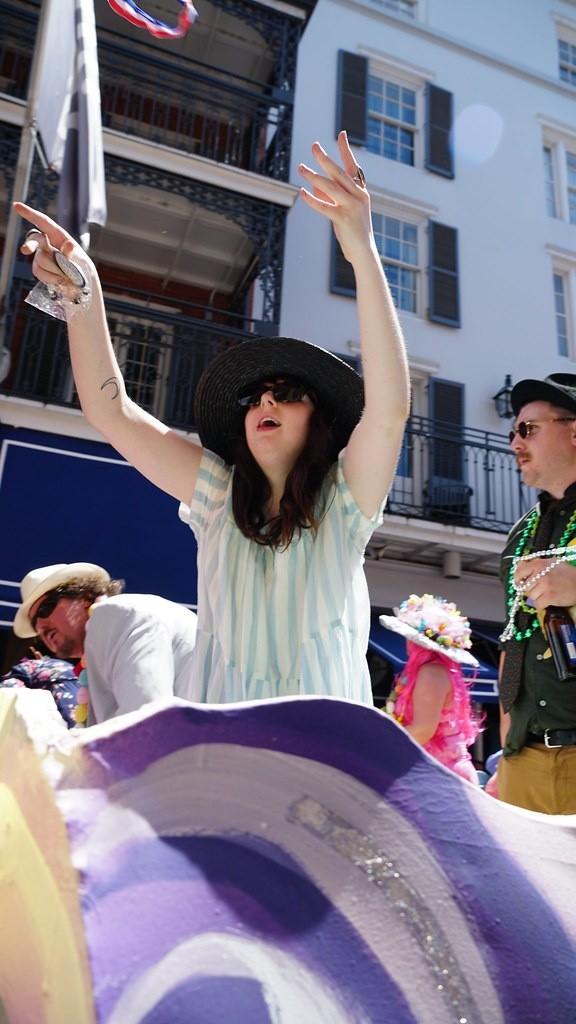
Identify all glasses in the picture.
[31,588,75,633]
[508,417,575,443]
[237,379,321,408]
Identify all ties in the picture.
[498,491,576,715]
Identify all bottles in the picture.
[543,604,575,682]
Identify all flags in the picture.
[31,0,108,251]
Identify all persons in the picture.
[496,374,576,817]
[374,593,479,790]
[12,130,411,715]
[12,560,202,727]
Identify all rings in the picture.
[347,166,366,181]
[25,228,48,251]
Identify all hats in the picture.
[511,373,576,415]
[194,336,366,466]
[13,562,111,638]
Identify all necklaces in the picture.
[499,504,576,637]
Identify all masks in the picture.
[406,639,424,658]
